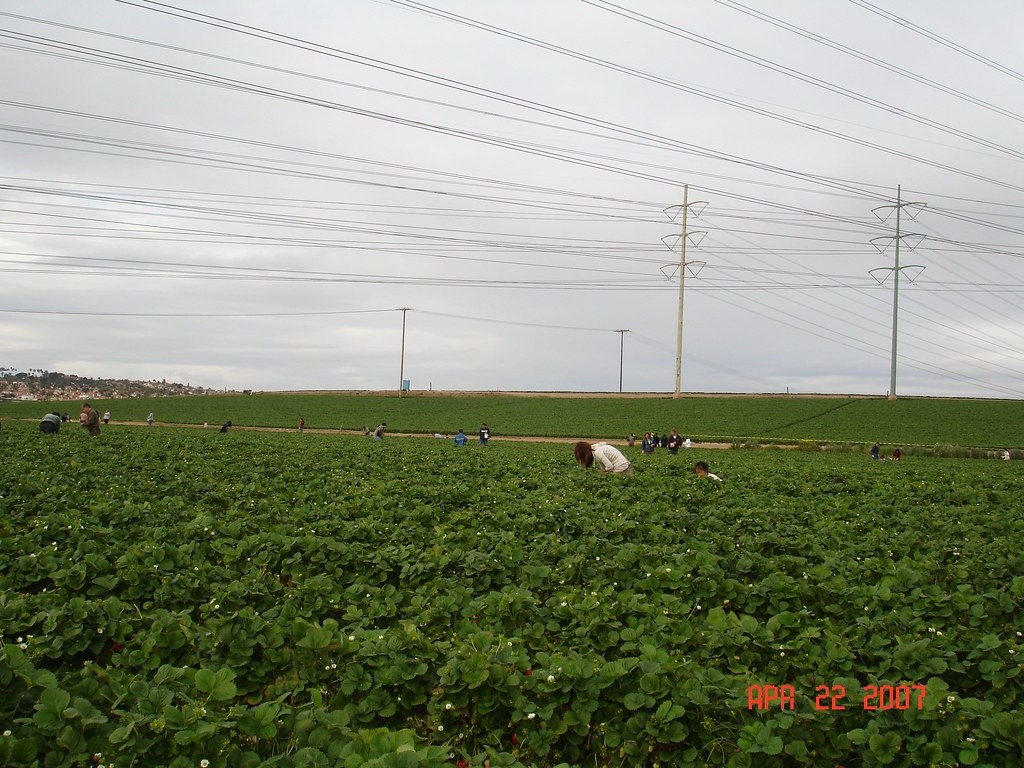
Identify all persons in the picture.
[37,402,388,441]
[433,422,1010,483]
